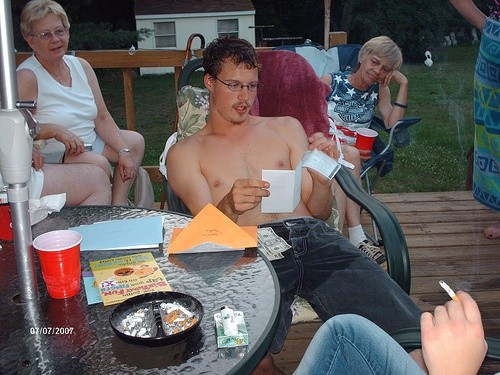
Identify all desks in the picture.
[0,205,281,375]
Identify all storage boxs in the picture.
[214,311,248,348]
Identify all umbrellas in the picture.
[183,34,207,66]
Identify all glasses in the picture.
[208,73,265,92]
[30,26,69,40]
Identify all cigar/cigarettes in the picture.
[438,280,460,300]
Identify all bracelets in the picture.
[394,99,407,109]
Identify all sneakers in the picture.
[356,239,387,265]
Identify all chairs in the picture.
[158,43,500,361]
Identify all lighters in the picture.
[219,306,238,336]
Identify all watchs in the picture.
[120,148,130,154]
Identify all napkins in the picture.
[28,166,66,226]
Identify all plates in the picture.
[109,291,204,348]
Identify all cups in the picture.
[33,230,83,299]
[0,203,14,241]
[355,128,378,160]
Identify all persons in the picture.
[1,0,144,208]
[167,37,489,375]
[447,0,500,237]
